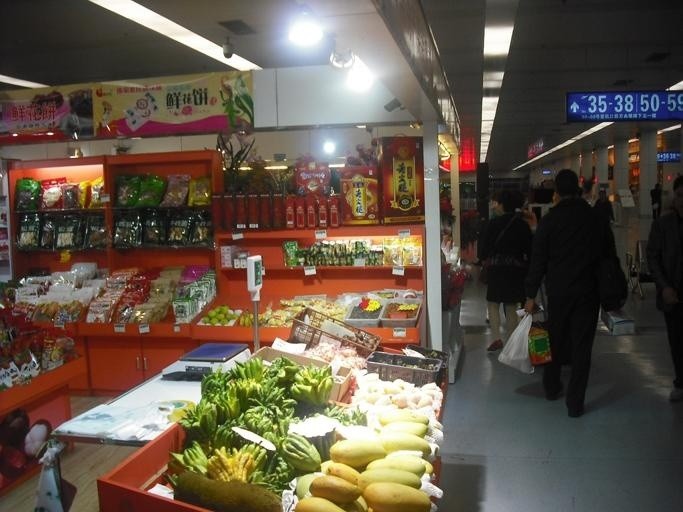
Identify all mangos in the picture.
[296,412,444,511]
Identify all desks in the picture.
[50,371,201,511]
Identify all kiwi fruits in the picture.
[342,329,379,350]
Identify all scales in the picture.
[161,256,263,379]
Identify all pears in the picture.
[360,373,434,413]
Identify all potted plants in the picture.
[215,128,255,193]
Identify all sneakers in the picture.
[546,381,563,399]
[669,386,683,401]
[486,340,505,354]
[567,405,583,418]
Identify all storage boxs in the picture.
[601,308,636,336]
[528,326,552,365]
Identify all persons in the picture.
[460,169,616,417]
[644,176,681,400]
[650,183,663,219]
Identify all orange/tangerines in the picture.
[201,305,238,327]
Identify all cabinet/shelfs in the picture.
[0,348,82,497]
[97,345,449,512]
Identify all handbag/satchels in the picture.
[602,257,629,312]
[477,257,491,284]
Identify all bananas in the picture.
[239,309,263,326]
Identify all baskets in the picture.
[365,344,450,388]
[288,308,382,359]
